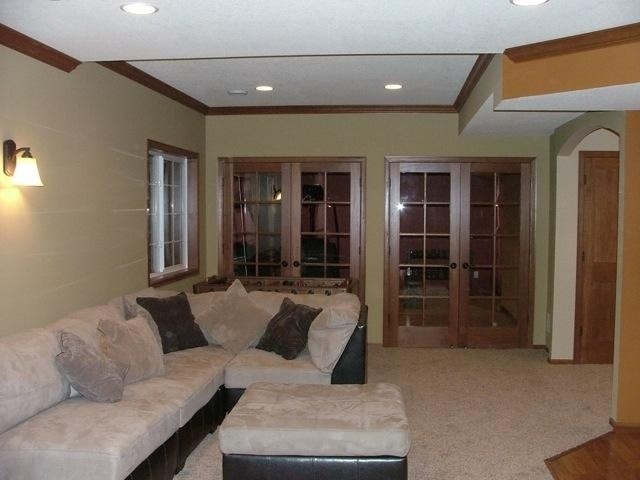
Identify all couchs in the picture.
[0,287,368,479]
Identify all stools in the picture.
[219,382,408,480]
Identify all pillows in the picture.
[96,314,175,384]
[253,296,322,362]
[195,281,272,355]
[123,296,163,360]
[307,293,362,373]
[136,291,210,354]
[56,326,123,402]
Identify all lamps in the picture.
[3,140,44,187]
